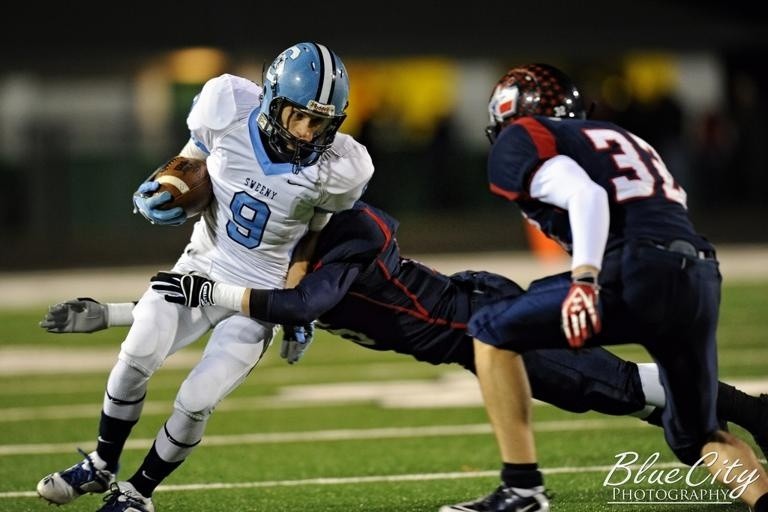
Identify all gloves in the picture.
[149,269,217,308]
[560,273,603,348]
[279,321,316,366]
[38,296,108,334]
[132,183,187,228]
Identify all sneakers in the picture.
[437,483,552,512]
[96,479,156,512]
[36,450,116,508]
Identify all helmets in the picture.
[257,42,350,168]
[484,63,587,147]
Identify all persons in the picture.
[33,40,374,512]
[38,198,767,463]
[436,61,768,512]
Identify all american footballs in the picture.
[151,157,213,218]
